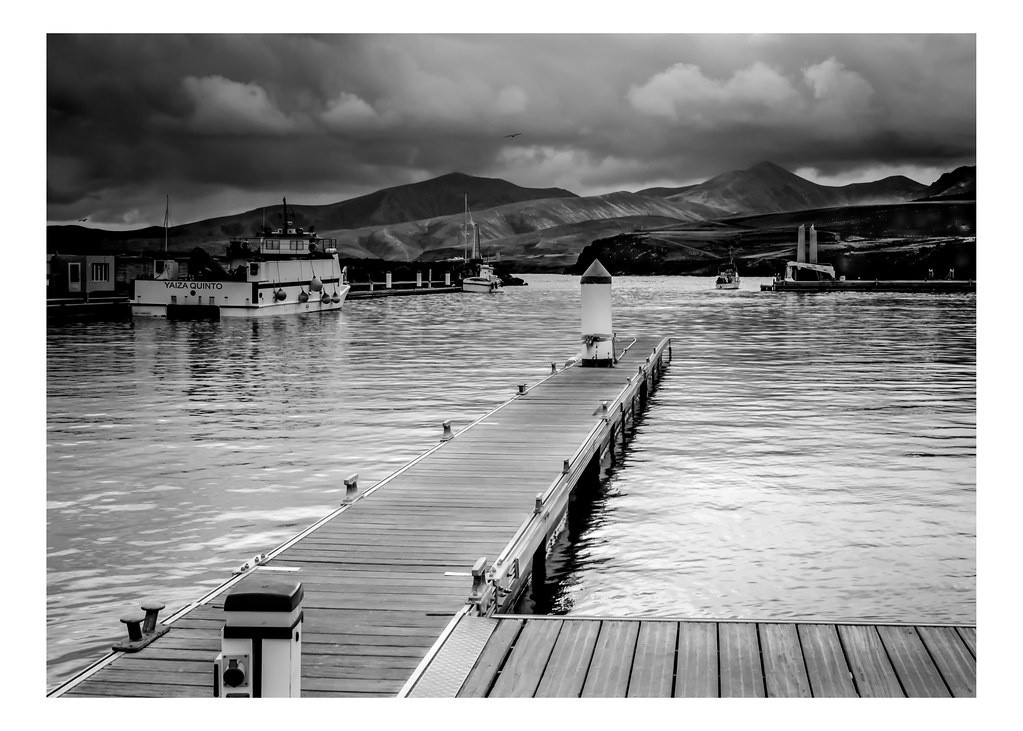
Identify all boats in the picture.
[715,250,740,289]
[118,193,351,322]
[461,264,504,294]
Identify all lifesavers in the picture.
[491,281,504,289]
[308,242,317,251]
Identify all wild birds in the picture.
[504,133,522,139]
[76,219,88,222]
